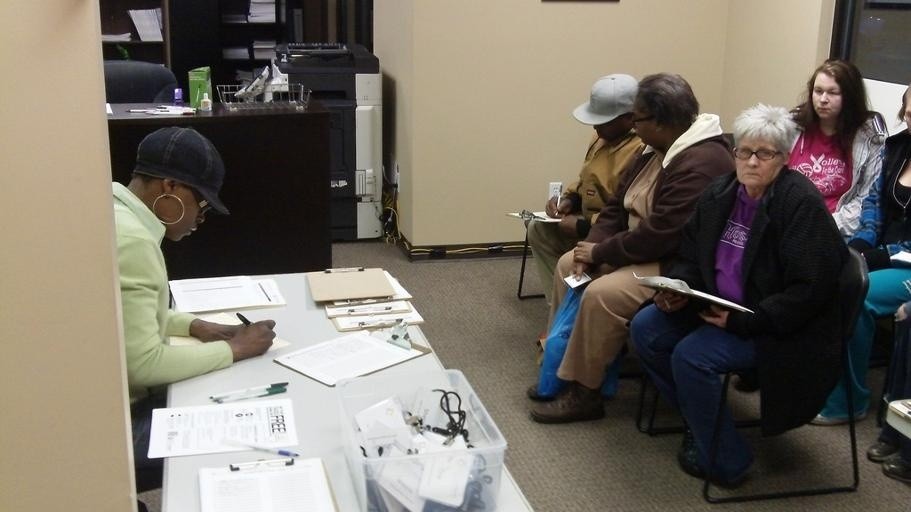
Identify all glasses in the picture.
[632,115,653,124]
[732,146,782,160]
[193,189,212,215]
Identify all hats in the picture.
[572,74,639,127]
[132,123,232,215]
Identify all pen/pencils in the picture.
[217,387,287,403]
[660,290,671,310]
[156,107,196,111]
[208,382,289,401]
[247,443,301,457]
[125,110,158,113]
[555,184,563,216]
[236,313,254,327]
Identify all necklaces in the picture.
[892,158,911,228]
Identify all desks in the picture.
[160,267,536,512]
[108,97,333,280]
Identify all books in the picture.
[221,0,277,83]
[102,9,166,42]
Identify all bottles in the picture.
[200,93,212,112]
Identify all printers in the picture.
[271,42,385,243]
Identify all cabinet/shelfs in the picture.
[99,0,287,101]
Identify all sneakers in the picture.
[883,456,911,482]
[528,382,557,402]
[810,408,869,427]
[865,438,903,462]
[529,382,606,422]
[680,426,697,453]
[679,447,739,488]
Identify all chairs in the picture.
[104,59,178,104]
[518,230,870,505]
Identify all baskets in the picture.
[215,83,311,111]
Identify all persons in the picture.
[813,88,911,424]
[110,127,277,489]
[787,56,888,236]
[526,72,734,424]
[630,102,849,483]
[526,73,646,367]
[869,321,911,485]
